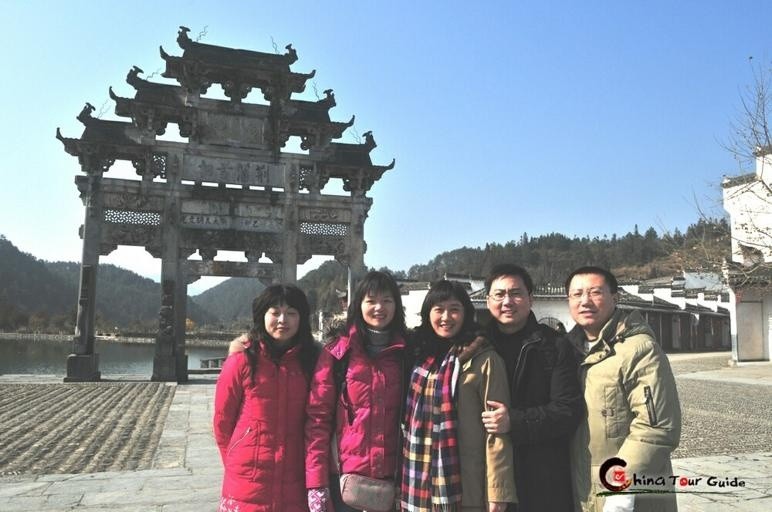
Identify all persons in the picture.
[306,268,411,511]
[407,279,520,512]
[470,261,581,512]
[564,265,681,512]
[213,284,323,511]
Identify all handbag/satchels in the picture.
[336,472,396,512]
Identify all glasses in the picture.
[567,289,613,298]
[487,291,530,300]
